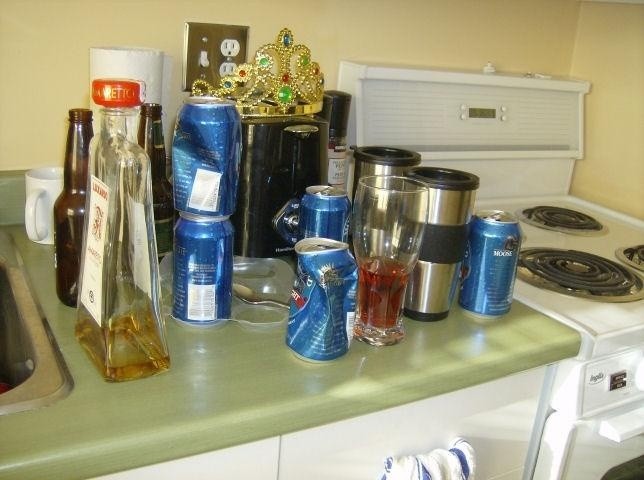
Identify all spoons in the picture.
[230,282,290,310]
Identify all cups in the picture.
[24,166,64,246]
[350,145,480,347]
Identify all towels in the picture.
[374,437,478,480]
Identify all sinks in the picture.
[0,232,74,414]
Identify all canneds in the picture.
[296,186,352,242]
[456,209,524,322]
[169,96,244,329]
[284,236,359,366]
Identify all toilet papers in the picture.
[89,48,173,139]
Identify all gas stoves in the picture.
[482,196,644,337]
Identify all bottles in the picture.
[323,89,353,196]
[54,78,177,383]
